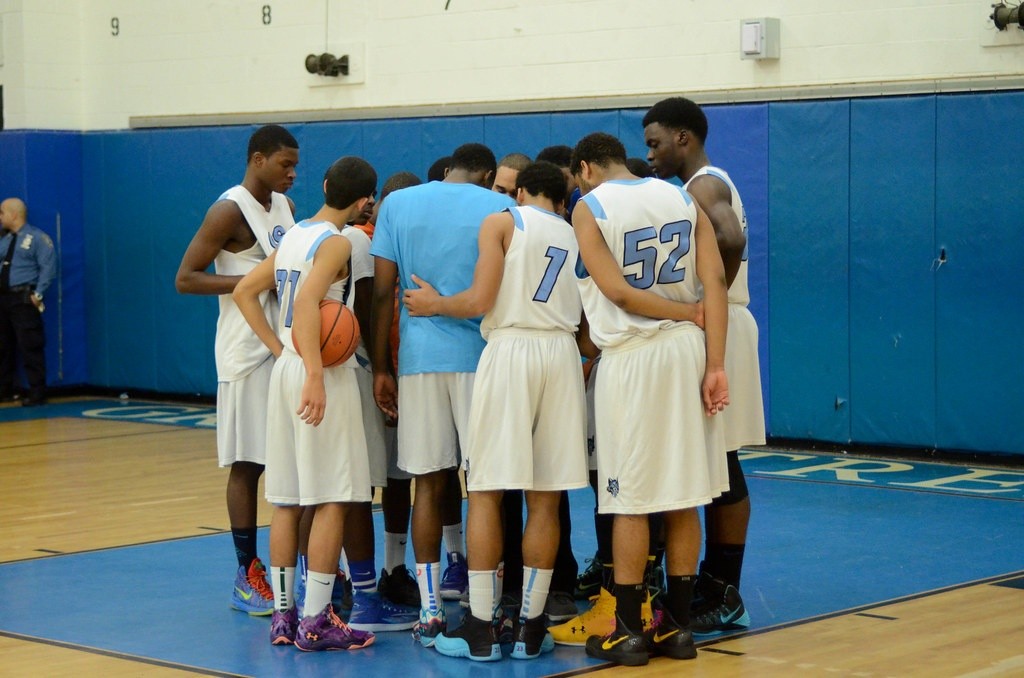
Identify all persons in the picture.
[0,197,58,408]
[176,122,517,653]
[403,96,766,667]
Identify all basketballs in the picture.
[290,299,362,369]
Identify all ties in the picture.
[0,233,17,291]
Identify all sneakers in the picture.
[377,564,421,609]
[332,579,356,613]
[270,605,300,645]
[546,587,617,645]
[509,613,554,659]
[645,621,697,658]
[544,591,579,622]
[585,631,649,665]
[689,585,750,636]
[412,621,447,647]
[434,610,502,661]
[575,551,606,599]
[347,590,421,631]
[440,552,468,599]
[294,603,375,653]
[231,558,275,615]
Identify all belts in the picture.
[9,286,26,292]
